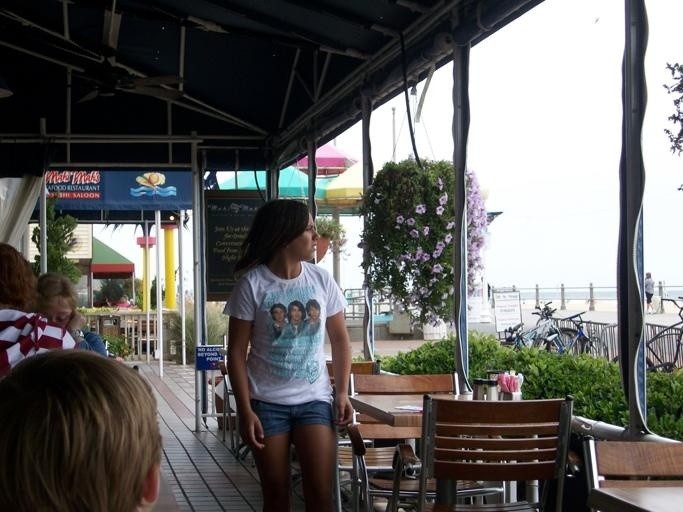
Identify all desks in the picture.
[590,487,682,511]
[349,389,503,428]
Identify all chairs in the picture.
[388,394,574,512]
[218,359,235,450]
[347,371,507,511]
[320,360,382,511]
[583,434,682,511]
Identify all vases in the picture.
[309,235,330,264]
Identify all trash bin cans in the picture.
[98,314,121,338]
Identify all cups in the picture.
[472,379,499,400]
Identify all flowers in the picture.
[358,155,489,331]
[314,216,349,260]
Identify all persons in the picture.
[269,299,322,340]
[644,272,656,315]
[1,242,162,512]
[226,200,354,512]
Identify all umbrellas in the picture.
[218,144,386,215]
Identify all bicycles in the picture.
[494,298,682,375]
